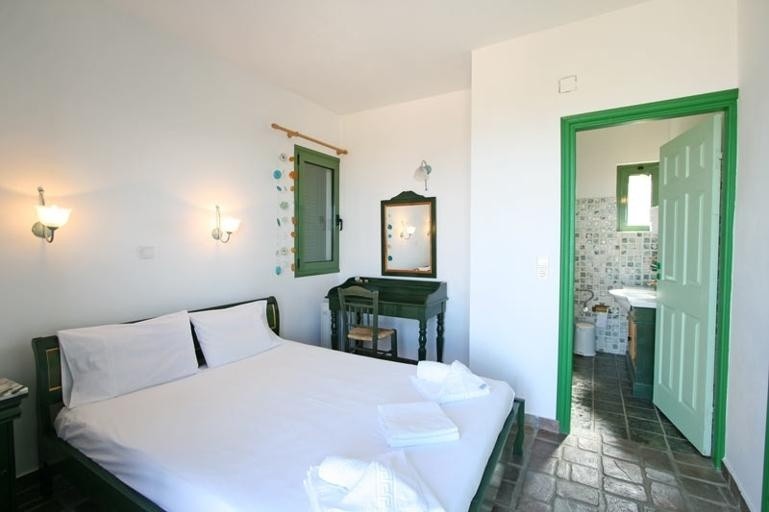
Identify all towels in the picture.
[303,356,488,512]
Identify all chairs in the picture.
[337,285,399,360]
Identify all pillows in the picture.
[54,300,286,410]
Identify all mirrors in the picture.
[380,190,437,279]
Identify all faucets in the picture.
[647,280,657,285]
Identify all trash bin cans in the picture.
[573,322,596,357]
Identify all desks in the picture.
[325,275,450,364]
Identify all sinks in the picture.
[607,288,656,312]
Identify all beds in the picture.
[31,295,530,512]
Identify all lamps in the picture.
[212,204,241,245]
[30,184,72,244]
[413,160,432,193]
